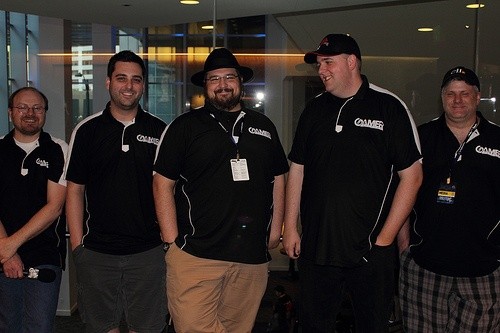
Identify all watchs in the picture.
[162,242,173,253]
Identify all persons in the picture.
[0,87,69,333]
[65,50,173,333]
[399,66,500,333]
[282,33,424,333]
[152,46,291,333]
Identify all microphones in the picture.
[22,268,56,282]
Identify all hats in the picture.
[191,48,253,87]
[440,67,481,91]
[304,34,361,63]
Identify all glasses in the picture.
[207,74,240,83]
[12,104,46,113]
